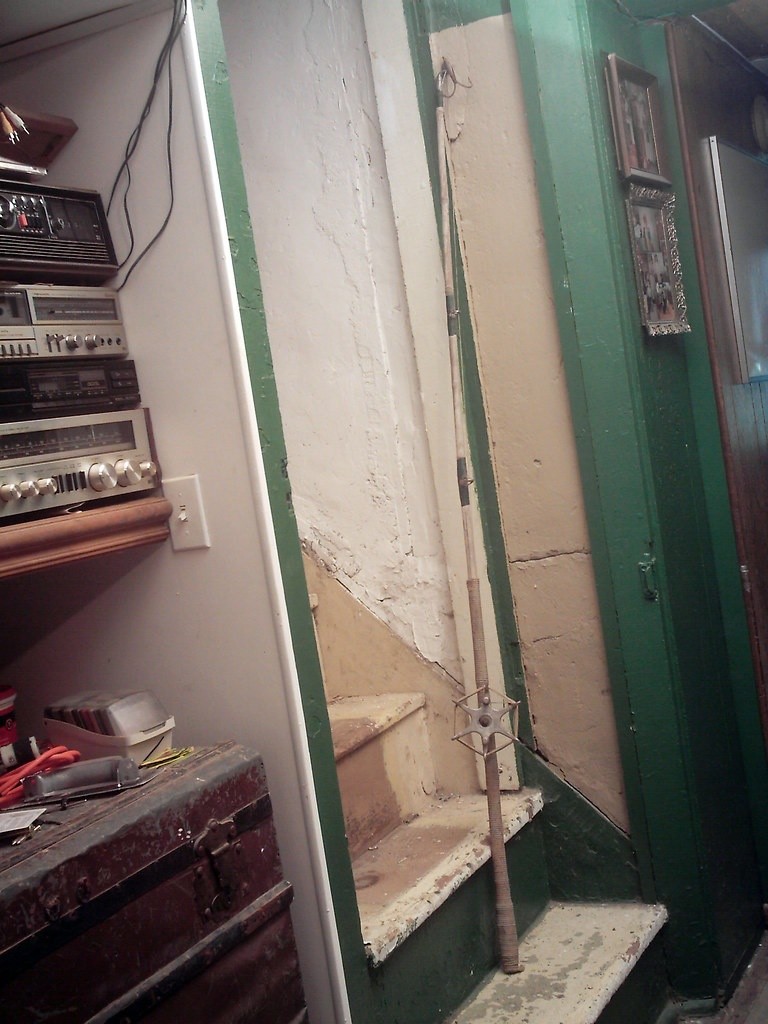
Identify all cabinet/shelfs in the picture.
[0,105,171,572]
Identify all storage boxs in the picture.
[0,736,310,1024]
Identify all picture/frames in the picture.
[625,181,692,337]
[604,52,672,188]
